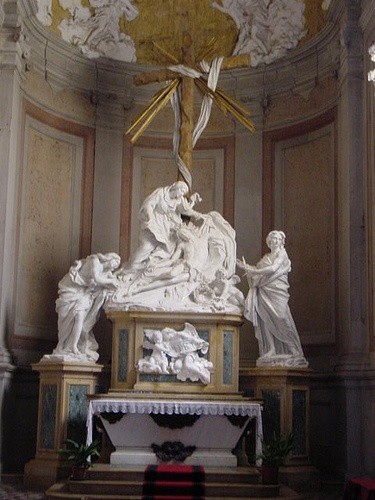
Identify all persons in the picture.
[210,269,247,307]
[125,226,210,297]
[118,181,203,281]
[236,230,304,358]
[55,252,122,354]
[161,323,213,385]
[142,330,171,373]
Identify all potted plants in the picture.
[251,431,297,485]
[56,438,103,480]
